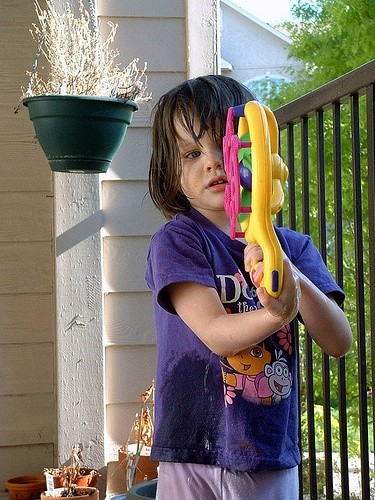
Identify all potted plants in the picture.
[17,0,152,176]
[38,379,158,500]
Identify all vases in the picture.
[4,473,46,499]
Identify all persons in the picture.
[142,73,355,500]
